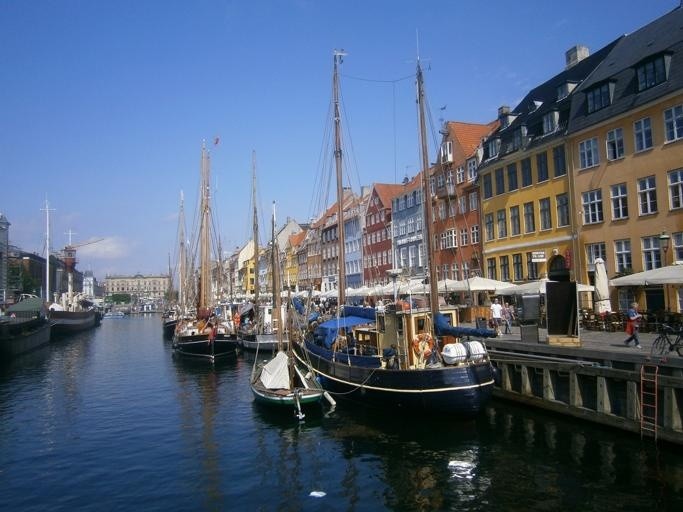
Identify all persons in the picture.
[489,297,502,336]
[623,301,641,348]
[500,301,514,335]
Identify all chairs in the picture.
[583,310,683,335]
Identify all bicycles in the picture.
[651,323,683,356]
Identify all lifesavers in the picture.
[414,334,434,357]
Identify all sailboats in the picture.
[214,147,313,352]
[171,138,238,365]
[297,29,497,416]
[163,190,197,336]
[248,200,325,407]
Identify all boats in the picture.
[0,295,125,358]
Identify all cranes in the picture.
[60,235,107,298]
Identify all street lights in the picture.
[659,230,672,311]
